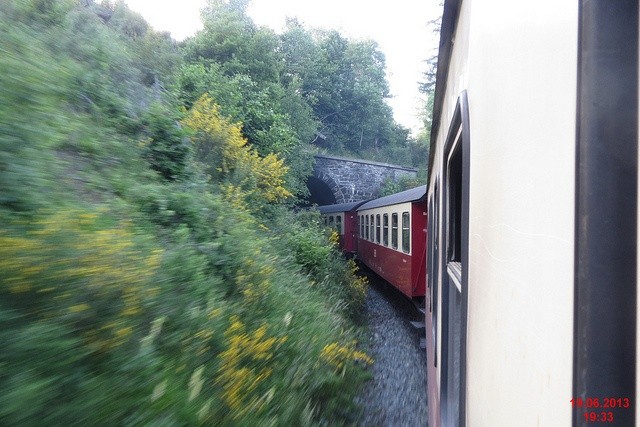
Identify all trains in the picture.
[294,0,638,426]
